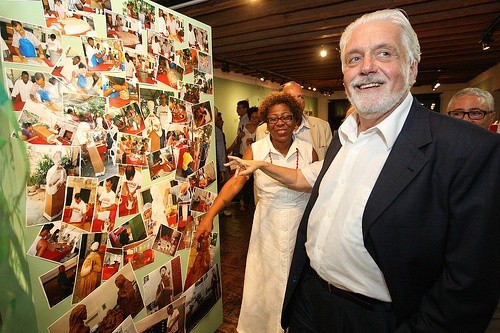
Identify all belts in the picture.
[307,272,401,313]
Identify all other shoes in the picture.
[221,210,232,216]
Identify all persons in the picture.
[214,106,233,220]
[2,1,220,333]
[226,100,253,212]
[284,8,499,333]
[446,88,495,133]
[226,107,259,212]
[196,93,325,333]
[342,106,355,120]
[256,81,332,163]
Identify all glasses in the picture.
[266,114,293,123]
[448,110,493,120]
